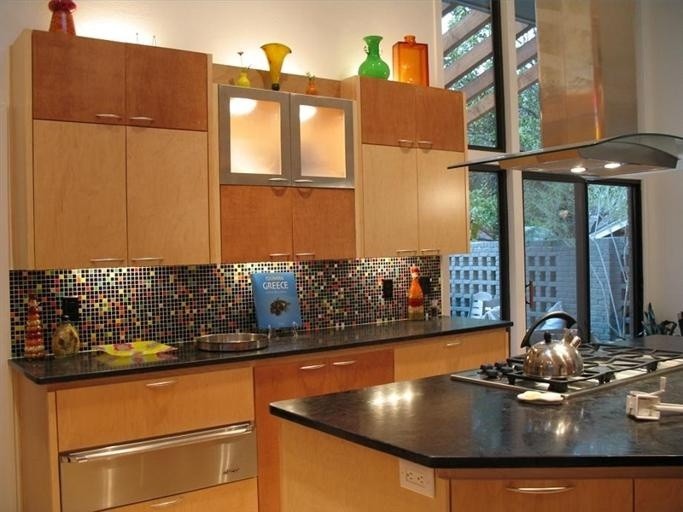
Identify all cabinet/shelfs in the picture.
[342,76,471,259]
[395,325,510,385]
[437,467,683,512]
[254,341,394,512]
[212,60,365,266]
[9,28,212,271]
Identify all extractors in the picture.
[446,1,682,181]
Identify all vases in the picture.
[358,35,390,79]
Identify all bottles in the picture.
[409,266,425,321]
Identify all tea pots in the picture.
[521,311,584,378]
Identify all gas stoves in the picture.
[449,343,683,401]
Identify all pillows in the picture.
[530,300,566,345]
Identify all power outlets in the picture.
[398,459,435,500]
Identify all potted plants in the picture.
[306,72,319,95]
[237,51,251,87]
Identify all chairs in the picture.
[521,311,577,351]
[483,298,500,320]
[467,291,494,320]
